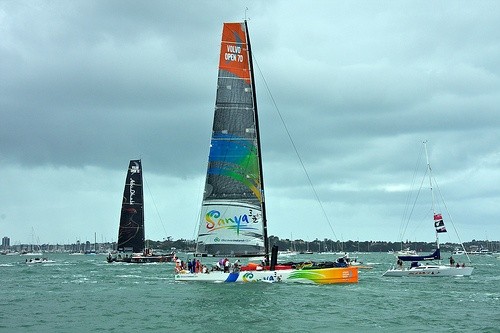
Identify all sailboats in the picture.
[173,8,360,285]
[381,138,475,277]
[106,158,176,264]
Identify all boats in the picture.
[451,247,493,257]
[317,250,350,256]
[387,242,417,256]
[299,251,313,255]
[21,257,56,264]
[275,250,297,257]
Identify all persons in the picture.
[396,259,403,270]
[174,256,268,275]
[448,256,466,267]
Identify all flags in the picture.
[434,213,443,220]
[434,219,444,227]
[436,226,448,233]
